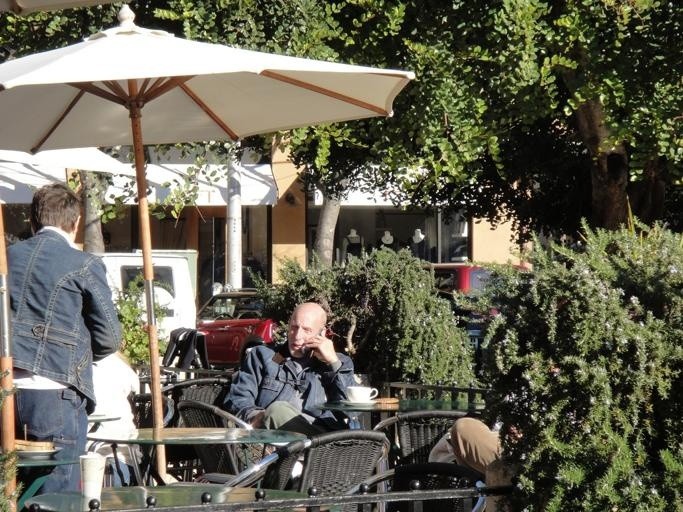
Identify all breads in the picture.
[13,439,55,451]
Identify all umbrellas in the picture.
[0,4,415,482]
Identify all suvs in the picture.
[193,287,288,372]
[422,261,532,351]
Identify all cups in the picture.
[345,386,378,401]
[79,455,107,497]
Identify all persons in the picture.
[6,180,121,494]
[230,302,354,491]
[451,417,523,512]
[340,228,431,264]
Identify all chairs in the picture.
[177,399,309,490]
[161,381,228,407]
[374,409,488,511]
[263,430,390,510]
[133,394,175,484]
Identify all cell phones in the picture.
[309,330,326,359]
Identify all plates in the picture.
[15,447,62,459]
[340,400,377,405]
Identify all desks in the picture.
[87,428,307,479]
[0,457,81,483]
[87,414,120,434]
[23,484,332,512]
